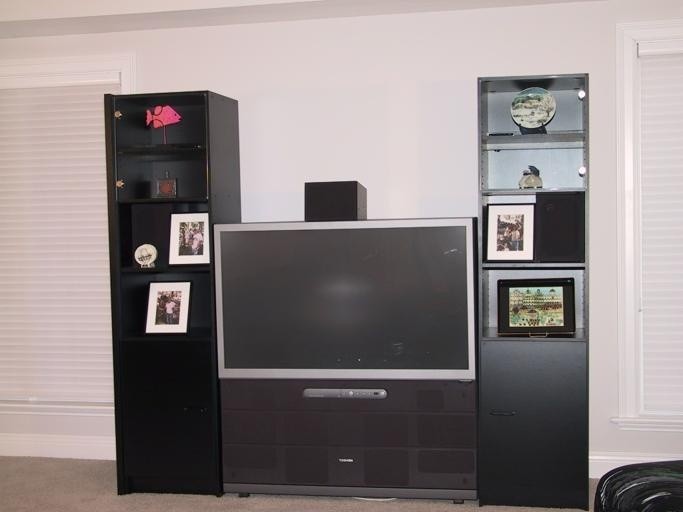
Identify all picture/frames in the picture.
[497,278,575,336]
[166,211,209,267]
[143,281,193,336]
[485,203,536,263]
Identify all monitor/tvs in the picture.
[213,217,475,379]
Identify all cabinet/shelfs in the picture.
[220,379,477,504]
[477,74,589,510]
[104,91,241,497]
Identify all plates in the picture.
[133,243,157,264]
[510,86,557,128]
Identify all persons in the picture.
[192,228,204,254]
[504,224,520,251]
[164,298,176,324]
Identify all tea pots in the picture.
[516,165,543,188]
